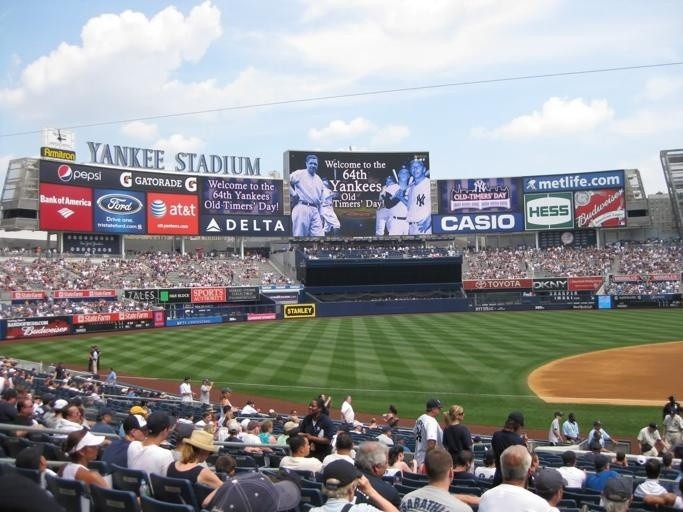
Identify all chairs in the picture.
[0,359,173,511]
[159,397,683,511]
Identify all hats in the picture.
[594,452,612,468]
[145,409,176,433]
[397,165,409,171]
[282,420,299,432]
[634,454,646,465]
[667,406,677,415]
[122,404,148,433]
[532,468,568,494]
[53,398,67,410]
[40,392,56,401]
[220,387,232,394]
[425,398,444,410]
[182,429,219,452]
[602,473,635,502]
[62,429,105,457]
[647,422,660,430]
[14,443,46,469]
[226,418,265,434]
[207,469,303,512]
[320,458,363,491]
[508,409,525,428]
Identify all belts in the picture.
[407,221,419,226]
[299,199,318,208]
[391,215,407,221]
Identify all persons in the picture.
[384,164,411,236]
[319,177,341,237]
[376,175,396,236]
[289,155,326,237]
[397,155,432,235]
